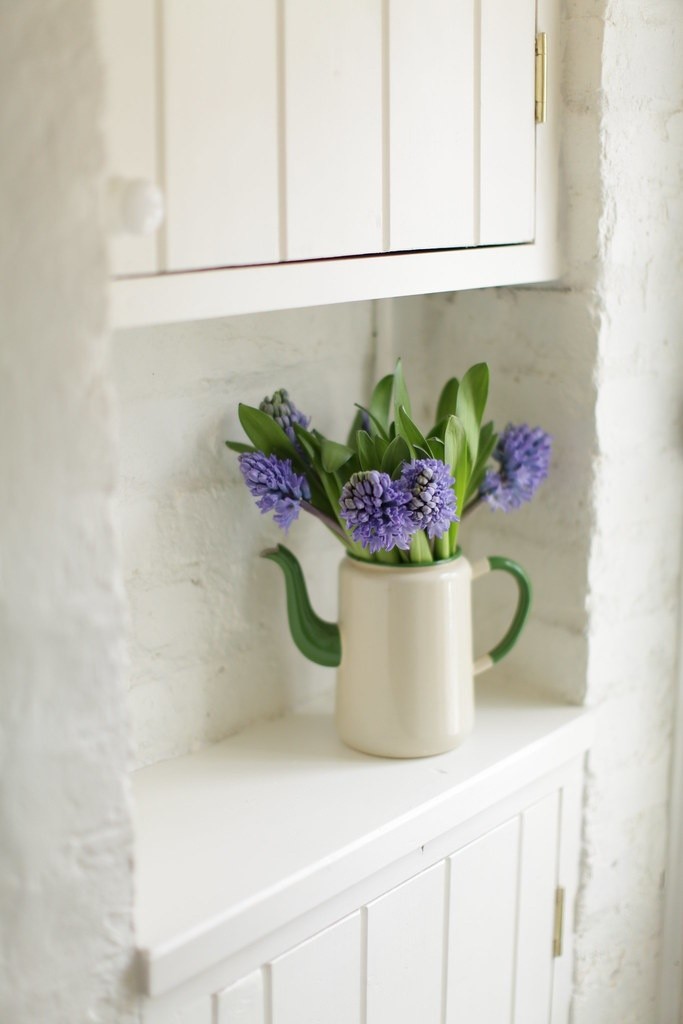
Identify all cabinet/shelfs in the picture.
[145,696,596,1024]
[107,0,575,332]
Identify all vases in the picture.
[243,539,552,763]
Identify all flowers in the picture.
[222,348,569,563]
